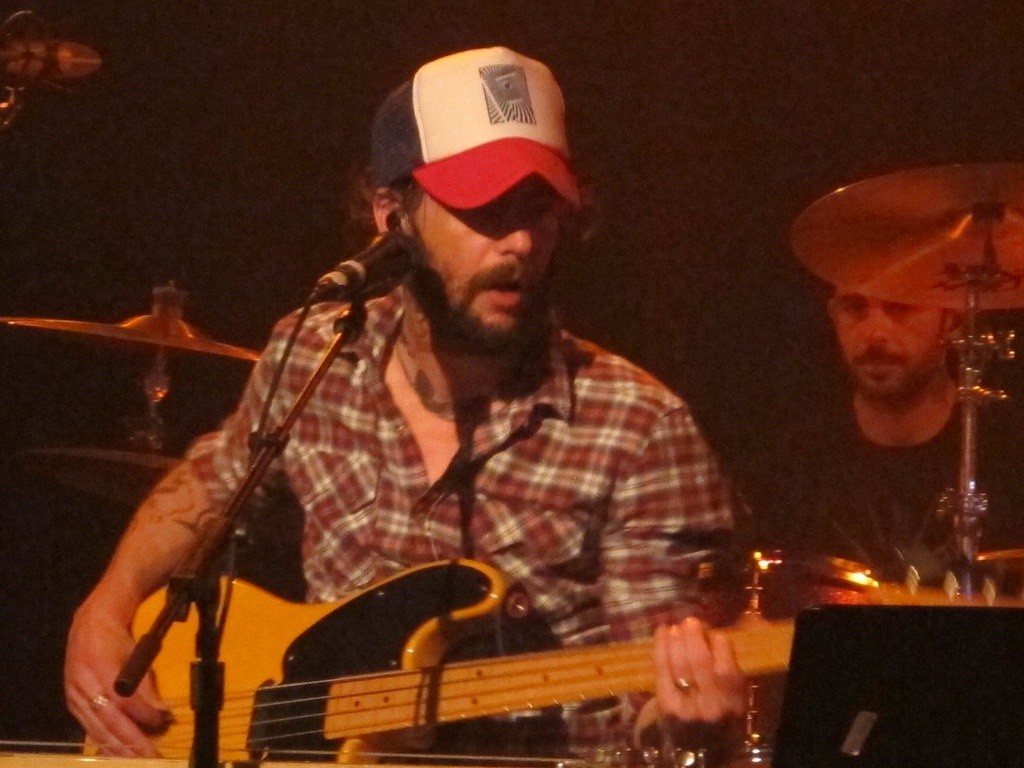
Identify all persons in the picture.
[59,41,754,758]
[747,288,1024,598]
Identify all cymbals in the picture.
[1,441,258,503]
[794,159,1021,313]
[0,314,261,370]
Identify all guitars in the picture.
[84,556,797,767]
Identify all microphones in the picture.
[305,230,419,303]
[0,40,103,81]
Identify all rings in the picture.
[89,693,112,714]
[674,676,695,688]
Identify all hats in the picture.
[367,45,585,214]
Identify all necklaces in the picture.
[393,333,545,421]
